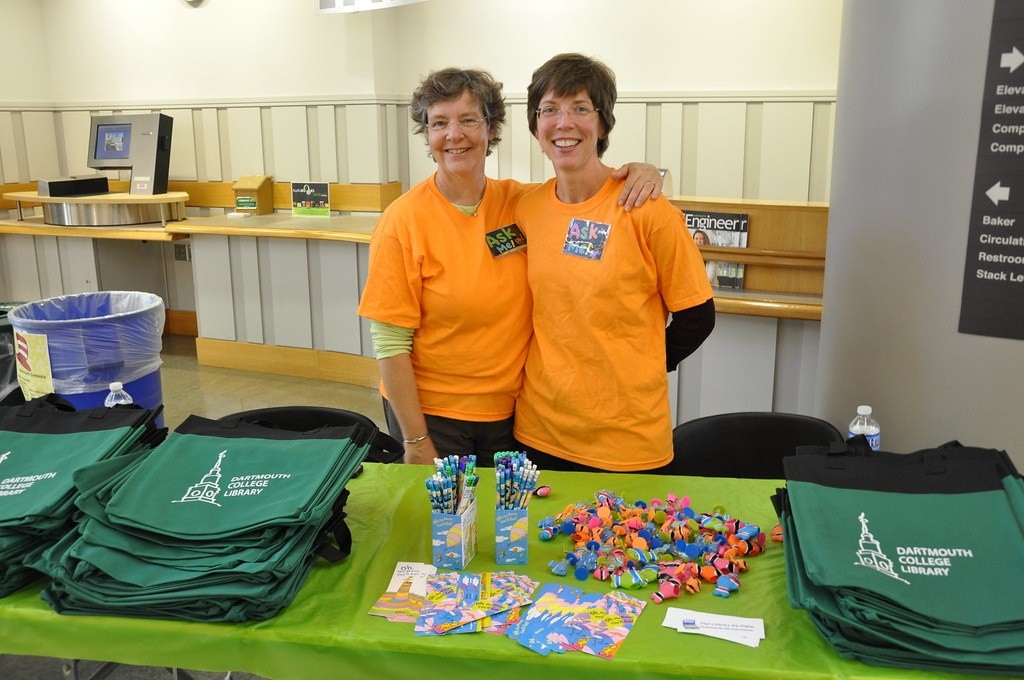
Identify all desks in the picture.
[184,208,381,390]
[702,288,822,321]
[0,461,998,680]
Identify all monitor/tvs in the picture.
[94,125,132,160]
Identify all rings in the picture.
[647,181,652,183]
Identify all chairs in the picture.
[217,406,377,428]
[671,412,845,480]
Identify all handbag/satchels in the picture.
[1,393,406,620]
[771,432,1024,675]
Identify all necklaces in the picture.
[437,180,483,217]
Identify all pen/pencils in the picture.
[447,477,452,514]
[505,470,511,510]
[434,482,445,513]
[429,482,441,514]
[425,479,437,513]
[518,467,525,486]
[521,470,541,510]
[518,470,534,510]
[494,451,537,473]
[512,469,529,510]
[460,476,480,514]
[500,471,505,510]
[451,467,456,511]
[455,476,473,515]
[443,479,448,514]
[495,473,501,510]
[508,472,521,510]
[432,455,476,506]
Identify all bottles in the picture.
[848,405,881,453]
[105,382,133,409]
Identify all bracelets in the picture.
[405,432,428,443]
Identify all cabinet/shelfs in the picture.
[0,192,190,341]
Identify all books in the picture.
[682,210,749,290]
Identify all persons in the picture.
[693,230,719,286]
[356,68,665,465]
[512,54,715,475]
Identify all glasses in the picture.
[536,105,601,122]
[424,115,488,133]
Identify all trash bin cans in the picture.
[7,290,164,428]
